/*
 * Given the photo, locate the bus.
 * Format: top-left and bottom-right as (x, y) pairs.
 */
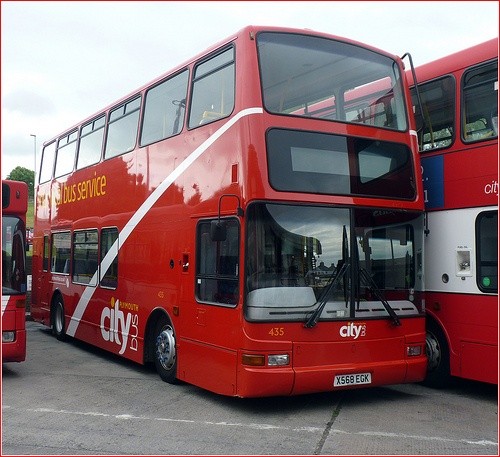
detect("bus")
(31, 24), (426, 399)
(288, 36), (498, 384)
(2, 179), (27, 363)
